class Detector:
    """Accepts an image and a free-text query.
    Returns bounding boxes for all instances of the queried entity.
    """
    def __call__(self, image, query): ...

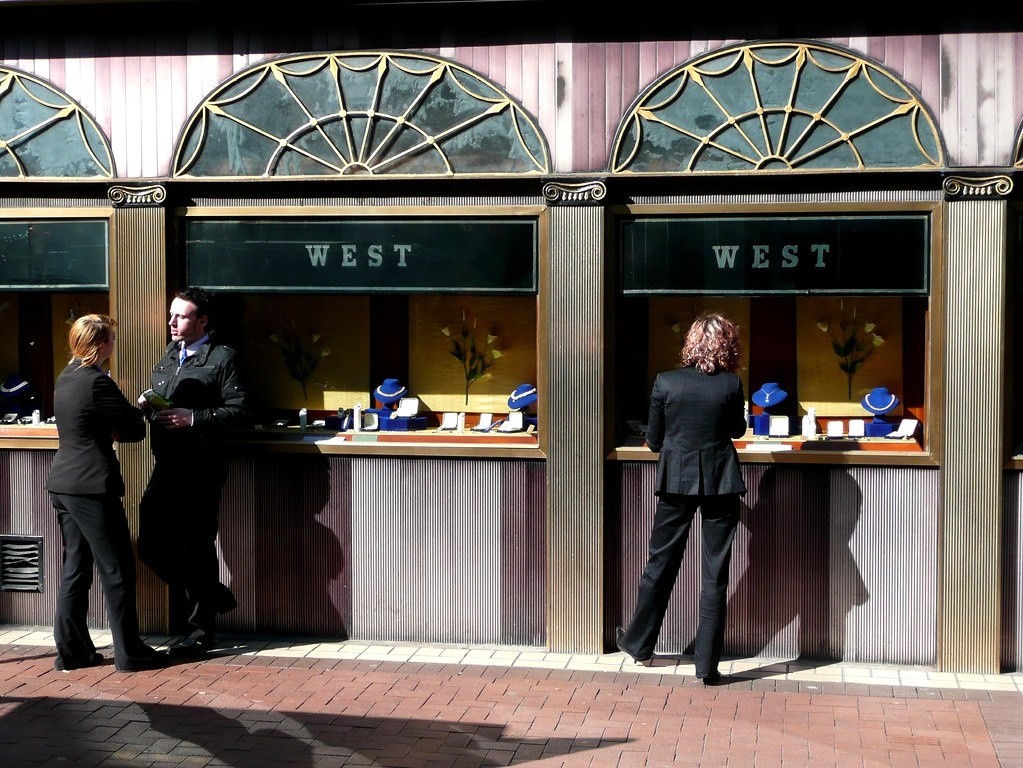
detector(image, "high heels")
[615,626,637,664]
[703,672,720,685]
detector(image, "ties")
[178,347,187,366]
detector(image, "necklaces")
[865,394,895,410]
[376,385,405,398]
[0,381,29,392]
[510,388,536,403]
[760,388,779,402]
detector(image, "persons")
[614,313,747,682]
[44,314,170,670]
[136,285,246,654]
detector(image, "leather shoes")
[214,584,237,616]
[168,632,214,657]
[201,635,203,637]
[86,653,103,665]
[144,651,167,670]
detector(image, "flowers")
[672,321,684,346]
[817,299,883,400]
[65,318,74,325]
[441,307,503,408]
[269,314,331,401]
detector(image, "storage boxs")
[497,411,523,433]
[396,397,419,417]
[768,414,790,438]
[440,413,458,430]
[884,418,920,439]
[361,413,380,431]
[849,418,865,439]
[825,419,845,440]
[0,413,18,424]
[470,413,493,432]
